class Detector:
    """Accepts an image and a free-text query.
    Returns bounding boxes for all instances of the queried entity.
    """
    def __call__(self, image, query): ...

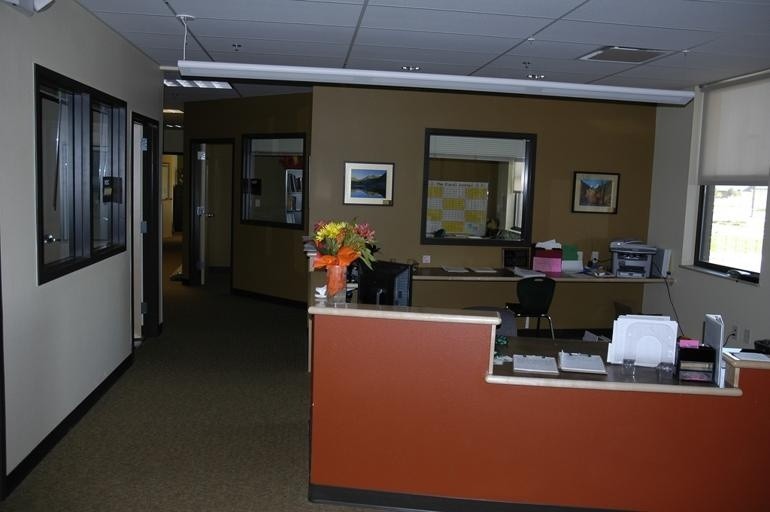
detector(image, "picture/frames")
[343,161,394,206]
[570,169,621,215]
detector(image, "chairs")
[505,276,558,338]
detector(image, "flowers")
[313,216,382,272]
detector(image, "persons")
[482,217,501,239]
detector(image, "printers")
[608,239,657,278]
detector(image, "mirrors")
[420,126,538,247]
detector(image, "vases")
[325,265,347,307]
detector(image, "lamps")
[176,11,697,108]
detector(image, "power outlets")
[731,326,738,341]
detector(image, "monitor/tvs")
[360,262,413,306]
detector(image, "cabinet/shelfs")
[285,169,304,223]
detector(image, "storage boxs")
[674,342,716,386]
[532,257,562,273]
[533,247,563,260]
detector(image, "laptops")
[503,247,530,270]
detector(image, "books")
[287,174,302,224]
[470,266,497,273]
[661,248,671,275]
[651,247,667,278]
[442,265,469,273]
[514,268,546,279]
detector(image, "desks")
[307,300,770,511]
